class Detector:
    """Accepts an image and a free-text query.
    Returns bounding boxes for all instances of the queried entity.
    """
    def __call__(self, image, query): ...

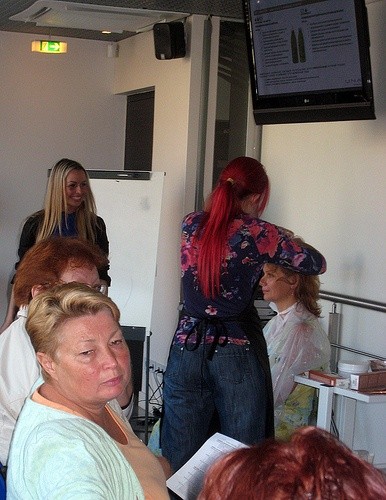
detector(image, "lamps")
[106,41,119,59]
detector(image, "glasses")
[83,282,107,295]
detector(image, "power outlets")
[148,359,165,379]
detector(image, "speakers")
[153,22,187,60]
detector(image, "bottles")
[337,359,369,379]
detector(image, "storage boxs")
[306,368,386,390]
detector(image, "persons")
[0,158,112,335]
[159,157,326,500]
[198,427,386,500]
[148,259,332,461]
[0,239,102,500]
[5,283,173,500]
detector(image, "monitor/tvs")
[242,0,377,125]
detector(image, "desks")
[293,373,386,434]
